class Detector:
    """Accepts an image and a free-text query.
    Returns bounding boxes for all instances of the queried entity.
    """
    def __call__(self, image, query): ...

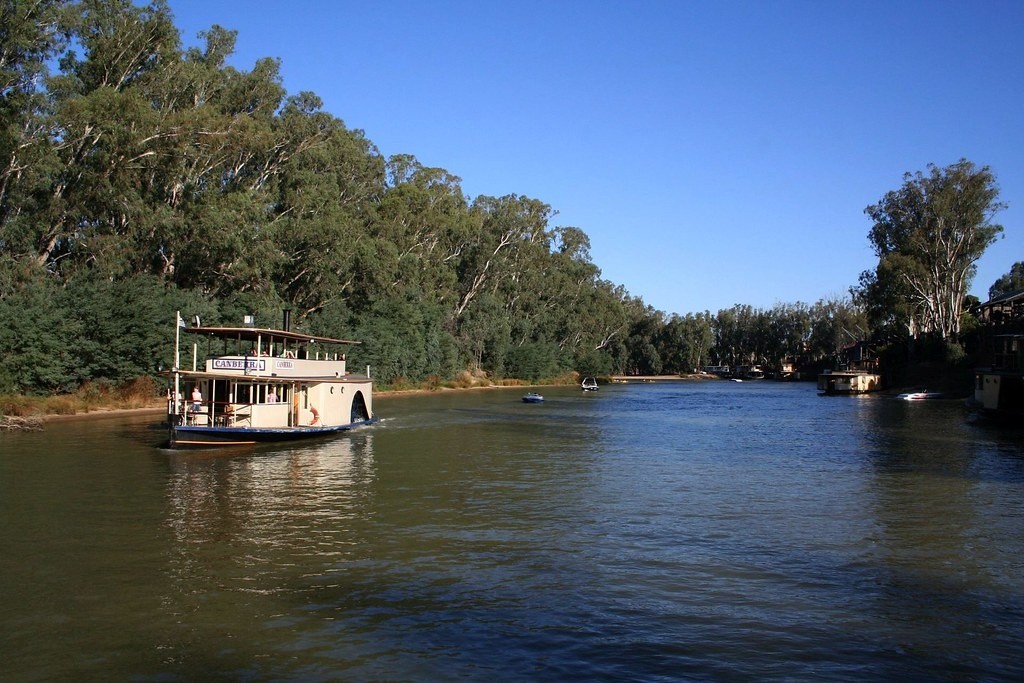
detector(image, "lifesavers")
[309,407,318,427]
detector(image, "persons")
[191,385,203,411]
[338,353,344,360]
[249,346,306,359]
[268,388,279,402]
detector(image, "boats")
[816,340,888,393]
[521,393,545,403]
[166,309,378,445]
[728,378,743,383]
[897,393,943,401]
[581,377,601,391]
